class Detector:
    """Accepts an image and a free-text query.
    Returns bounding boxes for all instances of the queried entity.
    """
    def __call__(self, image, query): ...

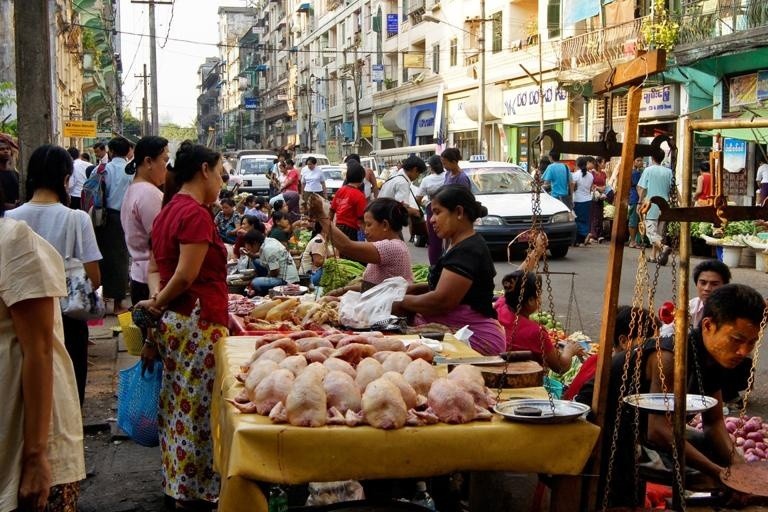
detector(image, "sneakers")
[578,233,605,248]
[630,239,672,265]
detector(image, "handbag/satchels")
[412,210,428,235]
[80,163,108,227]
[116,359,163,448]
[59,210,106,320]
[117,311,143,356]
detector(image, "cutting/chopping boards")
[450,360,545,388]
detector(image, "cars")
[408,154,577,259]
[293,144,437,199]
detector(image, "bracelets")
[144,339,159,348]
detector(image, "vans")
[223,150,278,194]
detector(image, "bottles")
[268,482,289,512]
[412,481,435,511]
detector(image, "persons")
[626,147,674,267]
[217,148,472,306]
[533,153,608,248]
[534,303,661,512]
[131,137,230,512]
[494,224,586,379]
[0,202,85,512]
[690,163,712,207]
[384,181,507,354]
[572,281,766,511]
[659,258,757,419]
[2,141,100,427]
[59,134,130,314]
[755,153,768,205]
[0,131,20,213]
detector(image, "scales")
[719,304,768,497]
[622,196,768,413]
[273,211,310,294]
[492,128,673,423]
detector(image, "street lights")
[422,14,484,148]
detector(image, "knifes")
[434,351,534,366]
